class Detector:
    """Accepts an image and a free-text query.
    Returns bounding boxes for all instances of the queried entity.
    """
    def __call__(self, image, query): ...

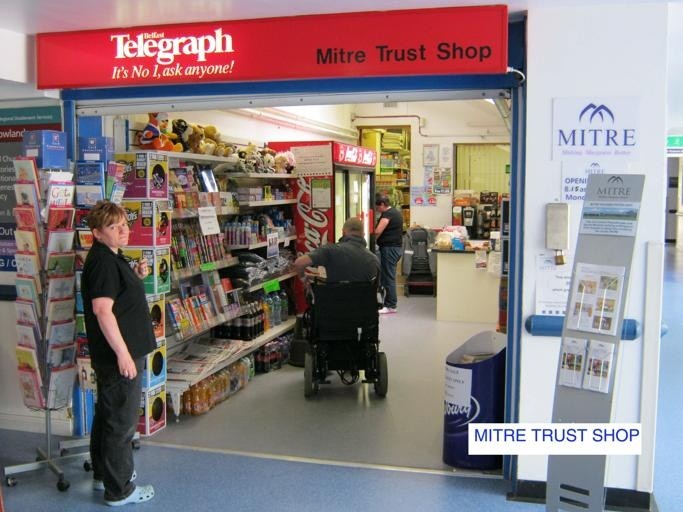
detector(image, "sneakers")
[378,307,397,313]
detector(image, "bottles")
[224,220,251,244]
[180,333,294,415]
[216,288,288,340]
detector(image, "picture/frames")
[423,144,439,166]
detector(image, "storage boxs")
[112,152,171,438]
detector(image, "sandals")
[104,484,154,506]
[93,469,137,490]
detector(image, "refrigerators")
[268,140,378,317]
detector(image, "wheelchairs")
[303,264,388,398]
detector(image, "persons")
[82,200,153,506]
[293,216,379,384]
[375,194,401,309]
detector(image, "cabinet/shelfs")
[114,119,309,424]
[359,125,411,235]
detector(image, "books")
[379,132,404,150]
[8,128,128,408]
[165,269,256,385]
